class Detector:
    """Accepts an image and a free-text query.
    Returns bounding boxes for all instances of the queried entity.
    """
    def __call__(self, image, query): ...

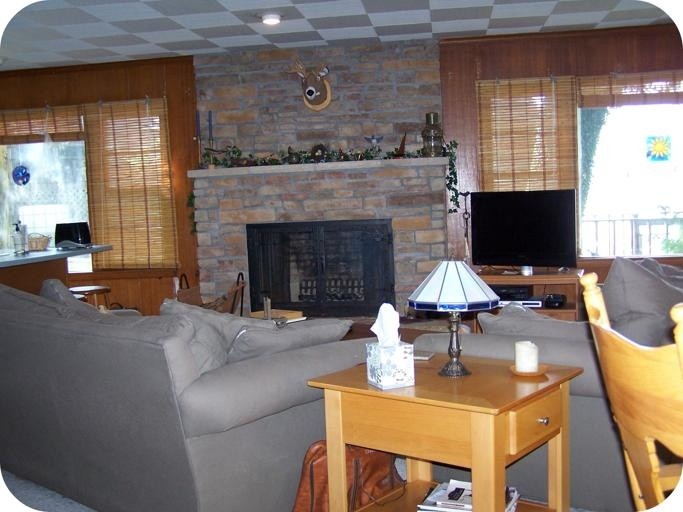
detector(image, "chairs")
[578,270,683,512]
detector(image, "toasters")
[54,222,91,249]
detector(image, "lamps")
[406,257,503,379]
[260,13,284,28]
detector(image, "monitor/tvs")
[471,190,576,276]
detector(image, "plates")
[509,364,546,376]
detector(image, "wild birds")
[364,134,383,148]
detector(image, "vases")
[421,112,445,159]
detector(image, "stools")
[69,285,111,310]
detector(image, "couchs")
[412,255,683,511]
[1,281,378,512]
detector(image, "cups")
[521,265,533,276]
[513,341,540,372]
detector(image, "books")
[416,479,520,512]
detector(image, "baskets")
[28,236,49,251]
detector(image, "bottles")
[422,111,443,158]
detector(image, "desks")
[1,243,114,296]
[306,349,585,512]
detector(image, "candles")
[513,340,542,376]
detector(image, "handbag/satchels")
[293,441,405,512]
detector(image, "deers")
[291,59,329,105]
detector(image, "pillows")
[158,294,277,354]
[476,254,683,340]
[226,317,356,364]
[39,274,99,320]
